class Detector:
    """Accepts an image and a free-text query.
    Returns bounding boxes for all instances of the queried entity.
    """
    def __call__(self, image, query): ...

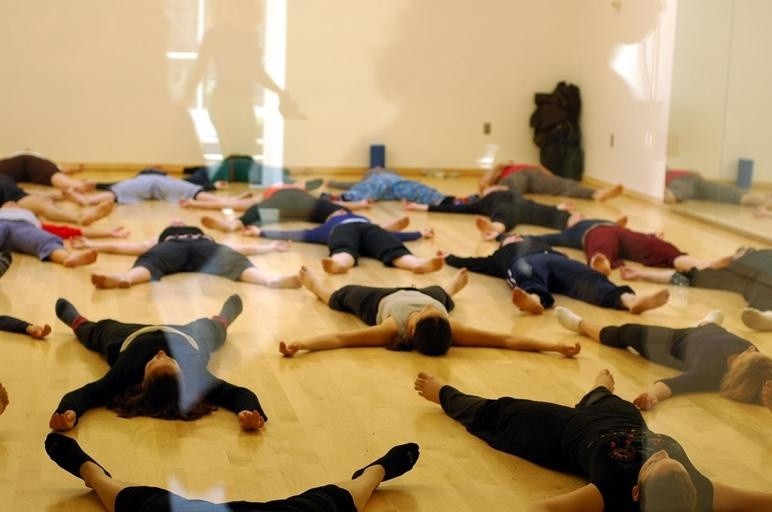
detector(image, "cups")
[370,144,385,169]
[736,159,753,187]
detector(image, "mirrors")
[662,0,771,240]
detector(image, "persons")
[0,200,130,267]
[0,383,9,416]
[751,205,772,218]
[479,213,733,277]
[414,369,772,512]
[243,208,446,275]
[181,156,323,192]
[0,175,113,226]
[179,184,374,233]
[402,186,584,235]
[551,307,772,412]
[63,168,253,206]
[50,294,268,430]
[663,167,765,204]
[435,234,670,315]
[479,160,623,202]
[45,433,419,512]
[279,266,581,358]
[0,155,96,193]
[619,248,772,333]
[69,220,302,288]
[321,165,480,205]
[0,250,51,339]
